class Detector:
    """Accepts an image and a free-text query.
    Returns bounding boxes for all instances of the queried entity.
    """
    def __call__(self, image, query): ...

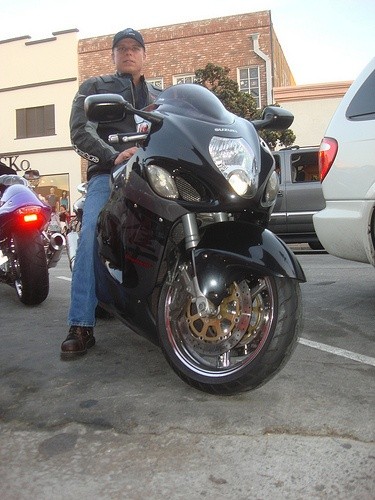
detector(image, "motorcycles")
[0,169,66,306]
[66,83,308,396]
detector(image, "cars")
[267,56,374,266]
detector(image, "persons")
[60,190,70,224]
[59,28,164,359]
[59,205,68,234]
[45,186,59,213]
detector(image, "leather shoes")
[59,327,95,359]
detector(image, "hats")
[112,29,144,48]
